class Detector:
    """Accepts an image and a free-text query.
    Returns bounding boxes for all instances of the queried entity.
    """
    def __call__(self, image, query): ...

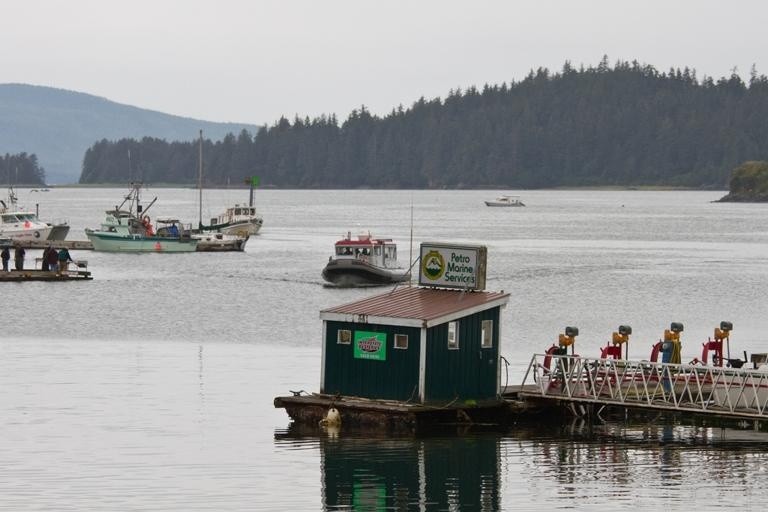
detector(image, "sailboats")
[182,129,264,251]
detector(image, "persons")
[1,244,10,271]
[15,243,25,269]
[40,246,72,272]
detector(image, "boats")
[321,228,412,287]
[484,193,527,207]
[533,321,768,411]
[206,203,263,234]
[86,177,195,251]
[0,181,71,239]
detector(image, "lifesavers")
[651,342,663,382]
[544,346,566,389]
[600,347,620,386]
[702,342,722,365]
[144,215,150,223]
